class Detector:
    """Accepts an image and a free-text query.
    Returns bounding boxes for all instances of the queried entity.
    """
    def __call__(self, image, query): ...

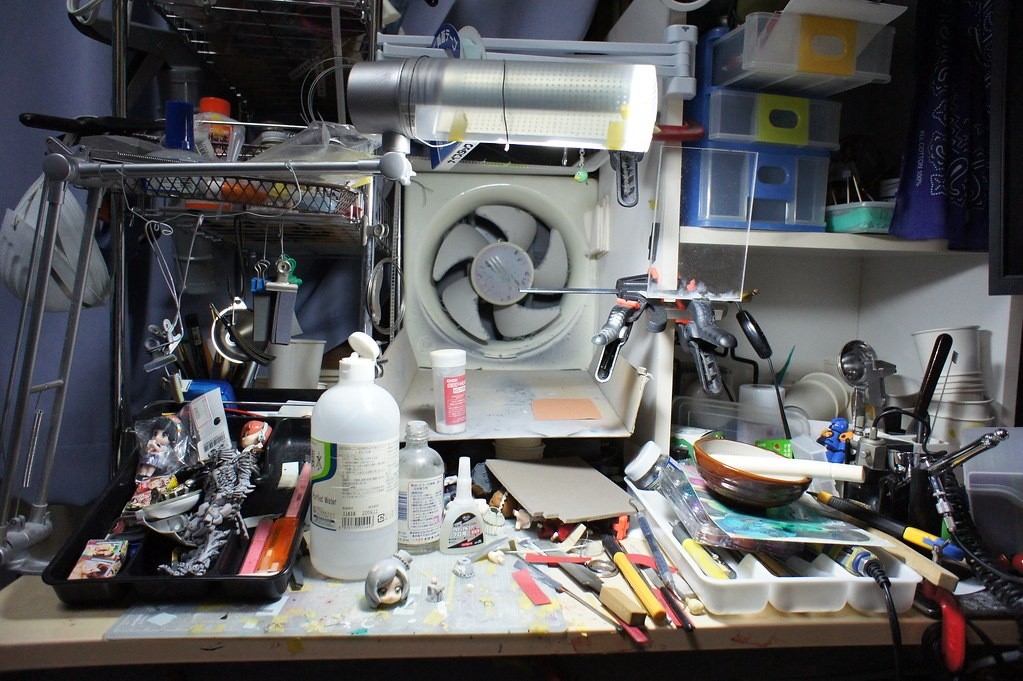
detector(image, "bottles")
[625,440,682,490]
[399,422,446,555]
[309,331,400,581]
[193,98,245,163]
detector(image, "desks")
[0,427,1023,681]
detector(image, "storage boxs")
[683,11,895,233]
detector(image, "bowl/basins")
[694,437,812,505]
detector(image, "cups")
[884,325,997,446]
[493,438,545,460]
[431,349,467,433]
[784,371,849,422]
[268,338,328,390]
